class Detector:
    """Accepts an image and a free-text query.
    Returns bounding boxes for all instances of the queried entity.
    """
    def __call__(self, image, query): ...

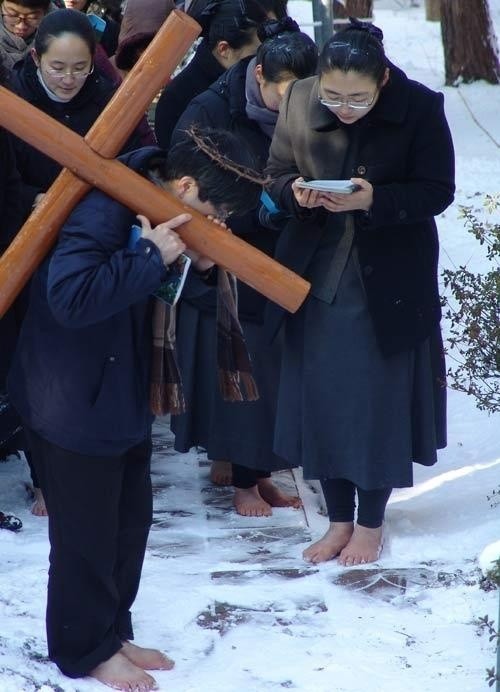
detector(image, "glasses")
[317,78,379,109]
[38,55,95,79]
[0,4,41,28]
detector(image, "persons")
[0,0,322,525]
[262,22,456,568]
[11,127,264,691]
